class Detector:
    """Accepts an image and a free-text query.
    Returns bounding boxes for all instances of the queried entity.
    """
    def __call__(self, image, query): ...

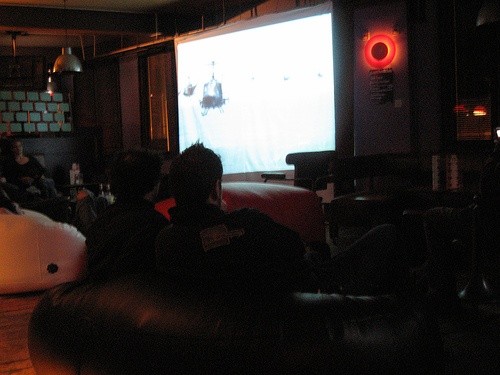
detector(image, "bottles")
[98,183,113,204]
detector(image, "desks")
[63,183,97,194]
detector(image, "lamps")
[51,0,84,75]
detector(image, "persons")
[83,161,169,287]
[0,137,56,214]
[151,143,313,312]
[324,187,436,325]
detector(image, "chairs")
[321,152,416,247]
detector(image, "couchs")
[28,273,447,375]
